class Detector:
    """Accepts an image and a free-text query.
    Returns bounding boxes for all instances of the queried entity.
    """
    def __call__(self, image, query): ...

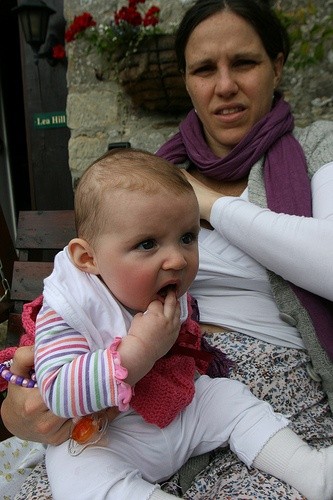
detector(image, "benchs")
[6,211,79,347]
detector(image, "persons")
[0,0,333,500]
[34,148,333,500]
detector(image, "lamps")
[12,0,59,68]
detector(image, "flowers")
[53,0,176,111]
